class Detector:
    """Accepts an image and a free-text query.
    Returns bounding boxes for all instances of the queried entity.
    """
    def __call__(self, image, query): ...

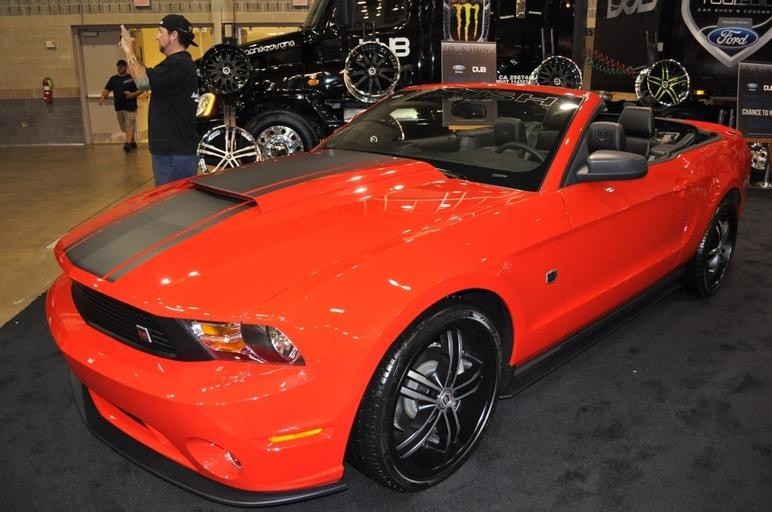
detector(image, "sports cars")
[37,80,758,510]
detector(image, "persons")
[118,14,201,188]
[99,60,146,152]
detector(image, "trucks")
[186,1,771,191]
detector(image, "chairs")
[572,122,626,171]
[481,117,529,159]
[534,105,578,150]
[617,107,658,157]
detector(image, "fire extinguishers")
[43,77,53,103]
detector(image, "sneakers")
[130,142,137,149]
[123,141,130,152]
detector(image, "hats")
[158,14,199,48]
[116,59,127,65]
